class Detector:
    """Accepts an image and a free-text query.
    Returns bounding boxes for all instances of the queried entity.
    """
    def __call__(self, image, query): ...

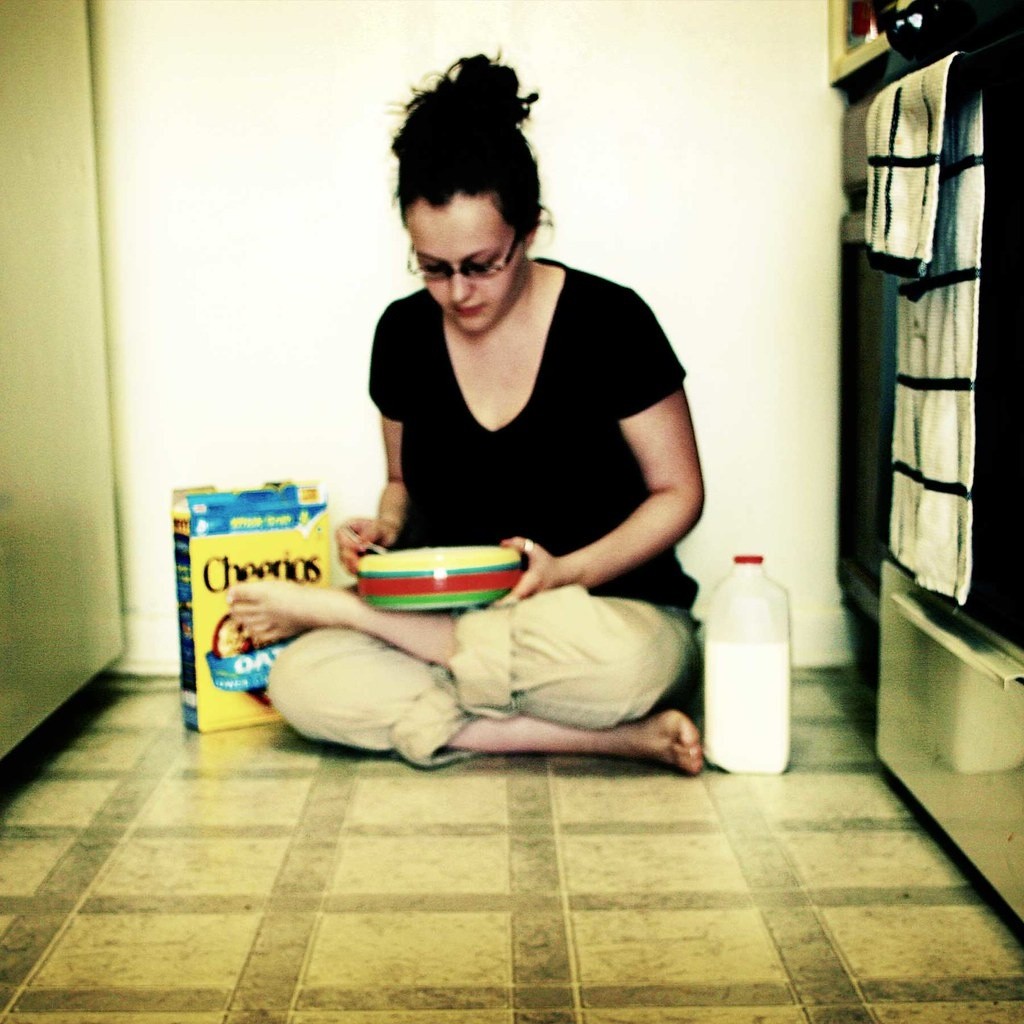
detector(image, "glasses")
[407,233,522,279]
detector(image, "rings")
[520,538,533,556]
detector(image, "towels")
[864,53,985,603]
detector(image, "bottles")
[705,555,790,775]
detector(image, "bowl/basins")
[356,546,522,612]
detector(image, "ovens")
[872,34,1024,946]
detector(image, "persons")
[228,53,706,776]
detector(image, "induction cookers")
[884,0,1024,59]
